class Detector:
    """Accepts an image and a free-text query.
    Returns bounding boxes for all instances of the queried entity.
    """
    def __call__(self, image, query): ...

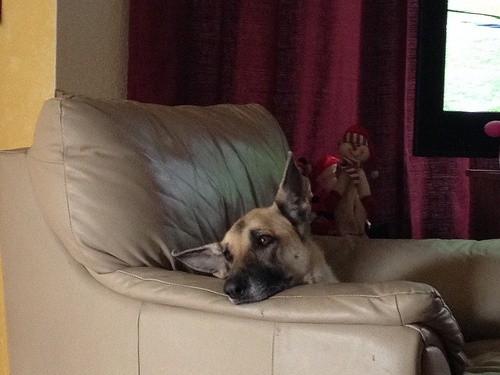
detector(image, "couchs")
[0,94,500,375]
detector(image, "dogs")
[172,151,341,305]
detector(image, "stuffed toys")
[314,127,379,236]
